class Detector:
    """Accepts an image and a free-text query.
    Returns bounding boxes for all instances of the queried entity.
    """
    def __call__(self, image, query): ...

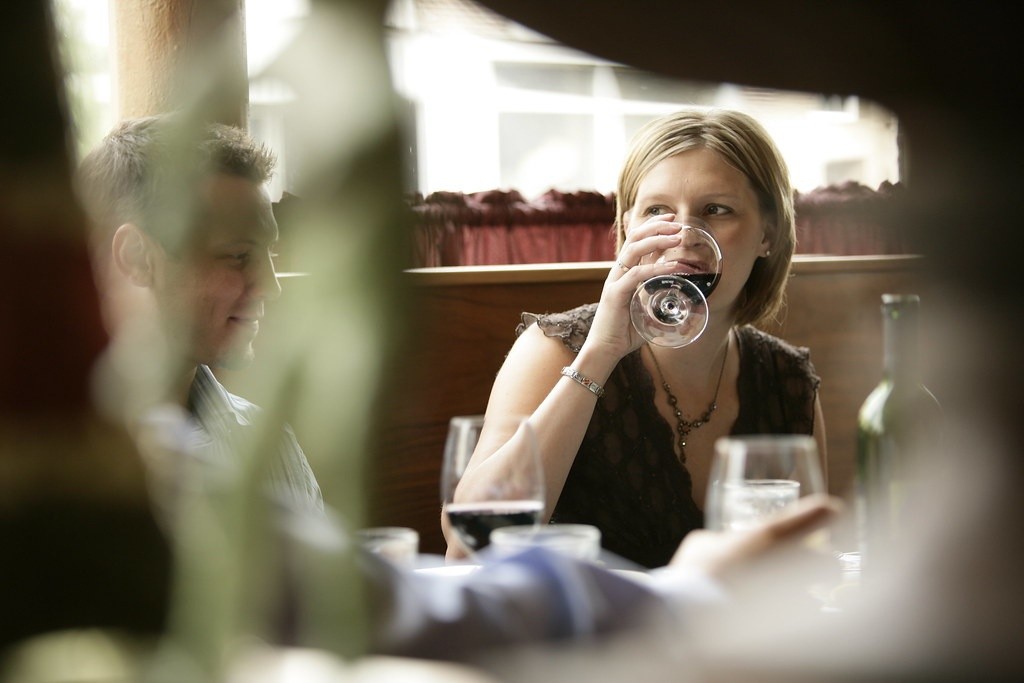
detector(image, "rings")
[617,260,629,273]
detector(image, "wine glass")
[630,226,724,349]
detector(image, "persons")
[441,108,832,565]
[80,117,845,660]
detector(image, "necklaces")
[646,336,731,465]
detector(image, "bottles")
[857,294,943,576]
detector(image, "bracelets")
[561,366,605,397]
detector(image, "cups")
[490,523,602,565]
[438,416,546,570]
[357,526,419,574]
[703,434,826,534]
